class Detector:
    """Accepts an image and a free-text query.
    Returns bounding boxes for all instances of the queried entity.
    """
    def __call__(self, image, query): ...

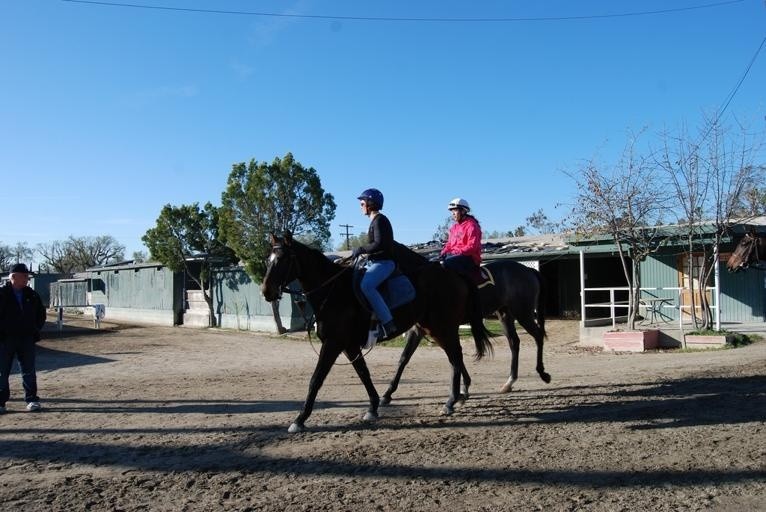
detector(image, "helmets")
[448,198,470,212]
[357,188,384,206]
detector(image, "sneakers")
[374,320,398,337]
[26,401,40,411]
[0,405,7,414]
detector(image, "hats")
[9,263,34,275]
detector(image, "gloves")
[352,246,365,258]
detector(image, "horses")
[378,240,551,406]
[726,231,766,275]
[259,227,504,433]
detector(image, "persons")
[353,188,400,337]
[0,262,46,414]
[440,198,481,284]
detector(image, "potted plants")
[684,330,742,351]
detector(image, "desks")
[639,298,675,326]
